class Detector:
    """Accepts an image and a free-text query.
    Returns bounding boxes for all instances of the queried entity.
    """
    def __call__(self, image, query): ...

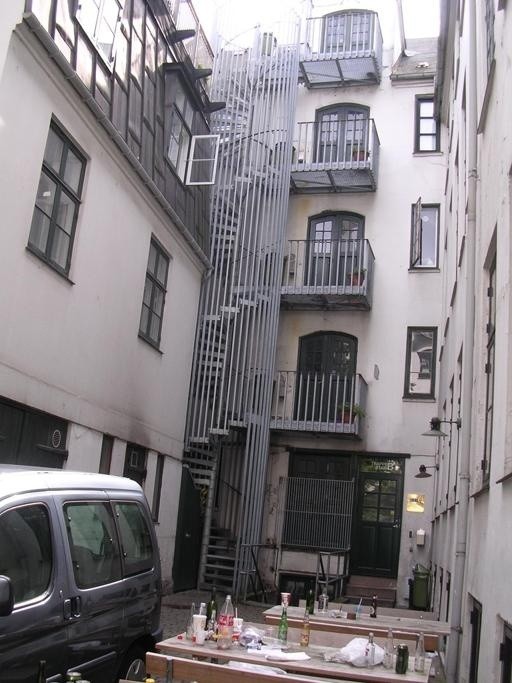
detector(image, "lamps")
[420,414,462,437]
[414,463,440,478]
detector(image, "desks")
[263,604,451,652]
[156,630,432,682]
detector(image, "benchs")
[298,600,440,622]
[266,616,439,654]
[144,652,310,683]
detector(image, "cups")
[232,616,243,632]
[353,604,364,619]
[194,625,209,645]
[280,592,292,608]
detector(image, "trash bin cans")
[412,563,429,609]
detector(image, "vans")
[1,460,165,683]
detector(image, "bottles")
[304,579,315,614]
[414,631,425,673]
[299,610,311,645]
[369,594,377,618]
[395,643,409,674]
[216,592,235,648]
[318,586,329,613]
[277,605,289,644]
[382,624,394,668]
[185,600,216,637]
[363,631,375,670]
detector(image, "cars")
[313,474,397,523]
